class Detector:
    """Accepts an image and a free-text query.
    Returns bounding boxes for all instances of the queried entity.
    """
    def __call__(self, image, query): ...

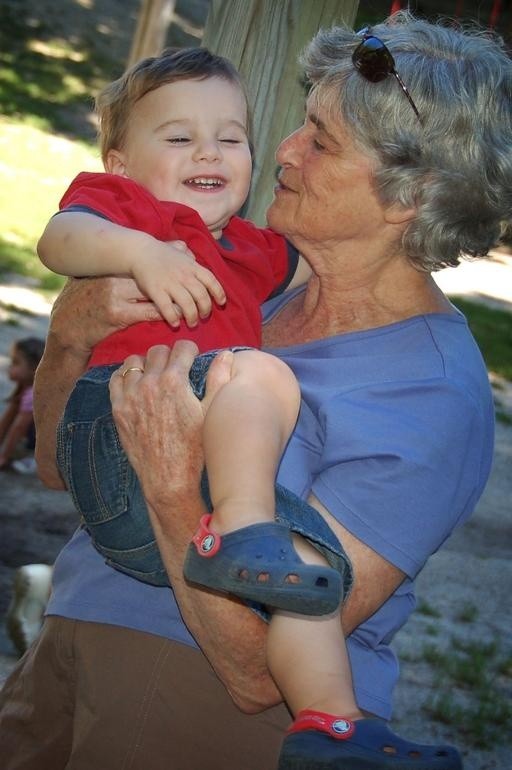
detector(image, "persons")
[3,11,510,768]
[0,336,49,476]
[35,48,467,768]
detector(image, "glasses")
[352,26,424,126]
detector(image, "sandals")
[280,710,463,770]
[182,513,344,616]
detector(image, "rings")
[122,366,144,378]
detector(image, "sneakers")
[12,458,37,474]
[6,563,50,652]
